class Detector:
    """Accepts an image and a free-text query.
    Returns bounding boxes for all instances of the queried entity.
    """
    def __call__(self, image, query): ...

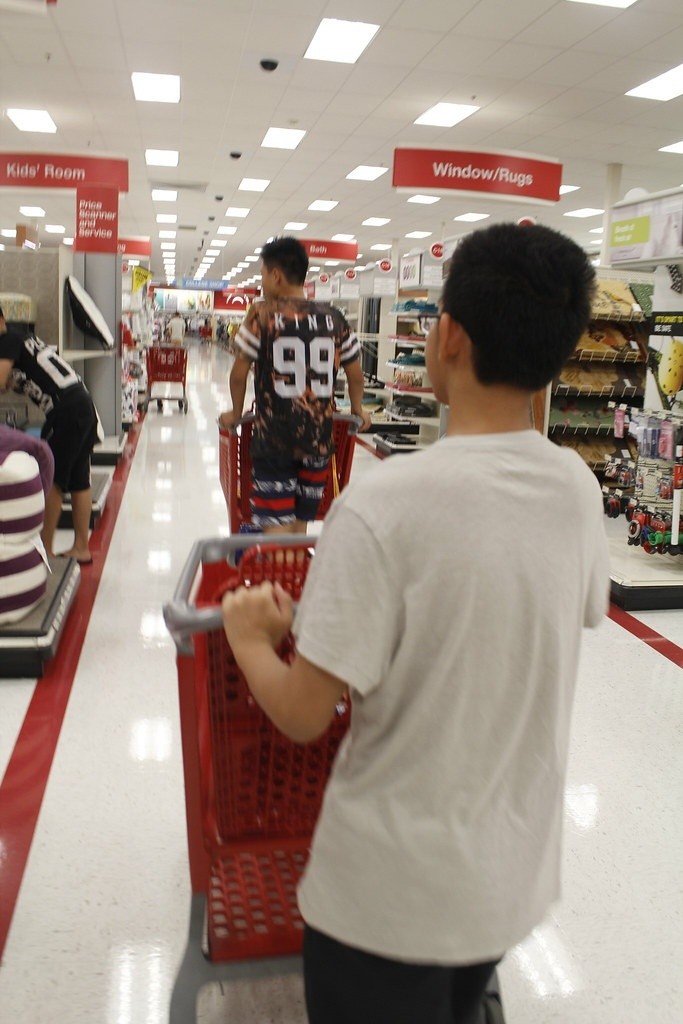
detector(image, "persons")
[0,308,107,567]
[168,312,187,347]
[221,221,612,1024]
[218,235,372,562]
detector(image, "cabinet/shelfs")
[57,246,168,466]
[531,268,652,499]
[319,284,447,461]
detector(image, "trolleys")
[167,537,359,963]
[144,340,190,414]
[219,406,363,539]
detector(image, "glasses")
[417,311,442,332]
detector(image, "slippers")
[56,551,94,564]
